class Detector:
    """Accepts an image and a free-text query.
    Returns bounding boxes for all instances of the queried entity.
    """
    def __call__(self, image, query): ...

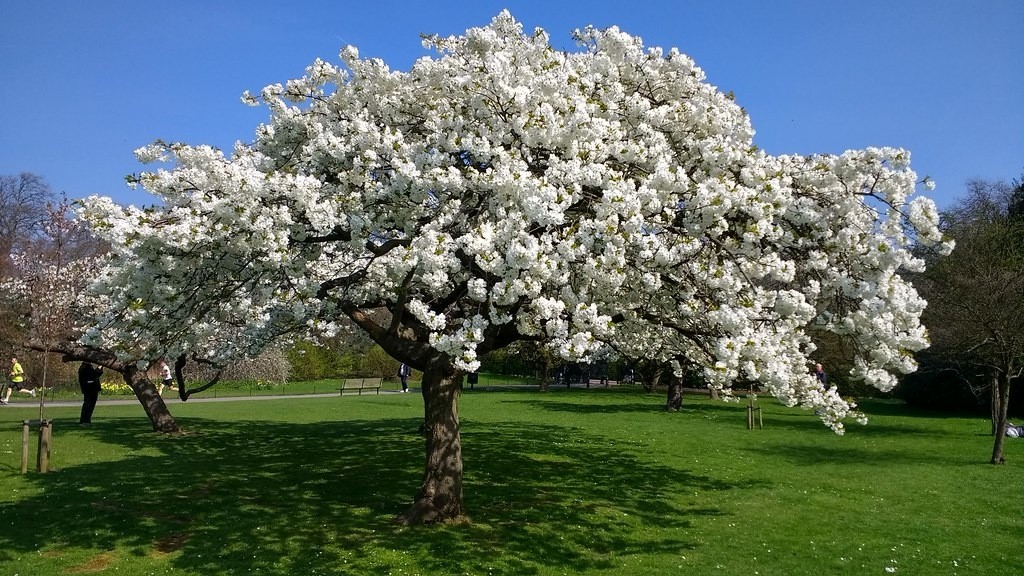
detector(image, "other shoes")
[0,399,8,404]
[406,388,408,392]
[400,390,404,393]
[32,389,36,398]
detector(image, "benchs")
[360,378,383,394]
[335,379,363,396]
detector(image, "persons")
[811,363,826,415]
[78,361,103,424]
[555,372,589,386]
[0,357,37,405]
[158,361,179,396]
[396,363,409,393]
